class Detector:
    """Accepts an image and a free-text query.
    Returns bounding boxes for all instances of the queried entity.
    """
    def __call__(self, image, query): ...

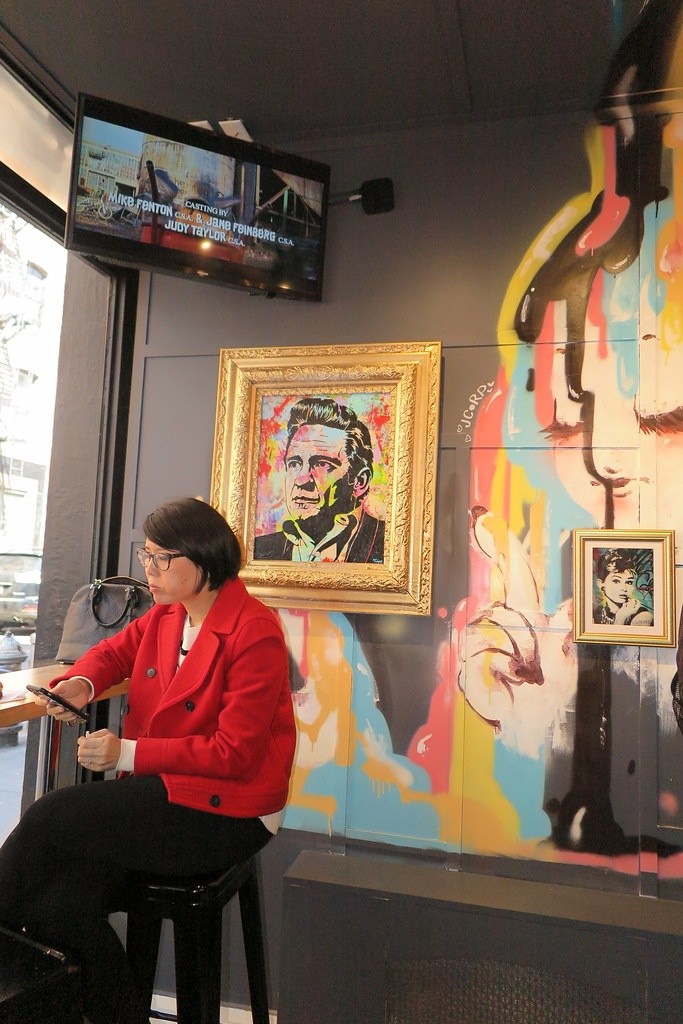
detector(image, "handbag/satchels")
[54,576,156,665]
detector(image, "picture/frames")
[572,529,677,648]
[209,341,442,616]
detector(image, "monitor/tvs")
[64,91,330,305]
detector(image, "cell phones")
[26,685,88,722]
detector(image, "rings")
[87,757,91,764]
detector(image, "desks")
[0,663,130,794]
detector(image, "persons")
[0,497,297,1024]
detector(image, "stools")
[127,853,268,1024]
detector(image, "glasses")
[137,547,187,571]
